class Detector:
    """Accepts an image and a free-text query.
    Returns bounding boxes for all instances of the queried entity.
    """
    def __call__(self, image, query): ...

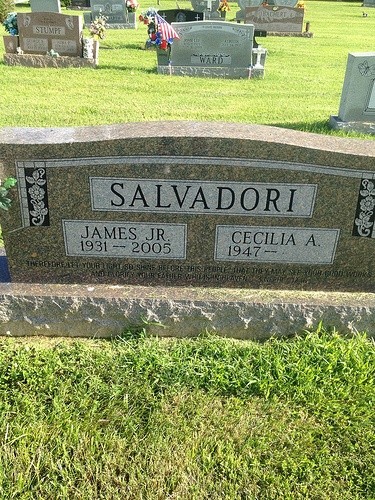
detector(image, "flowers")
[88,8,113,39]
[217,0,230,15]
[126,0,138,13]
[2,12,18,37]
[139,7,171,52]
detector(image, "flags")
[154,13,180,45]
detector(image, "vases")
[3,35,19,54]
[82,39,94,59]
[128,13,135,23]
[157,50,170,66]
[221,11,226,18]
